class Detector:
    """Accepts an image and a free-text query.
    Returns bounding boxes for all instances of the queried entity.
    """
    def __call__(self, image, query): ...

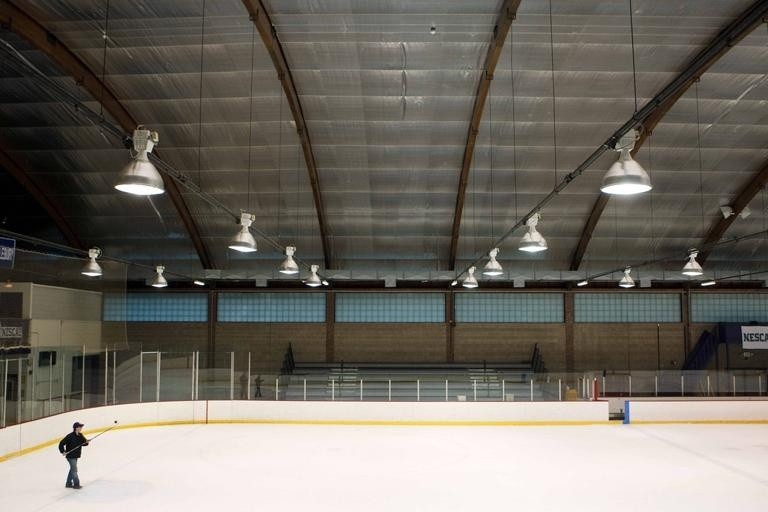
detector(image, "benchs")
[286,360,546,403]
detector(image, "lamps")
[113,125,322,290]
[79,247,171,290]
[739,207,752,220]
[460,119,655,292]
[720,206,735,220]
[617,248,705,289]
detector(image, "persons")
[254,373,264,398]
[58,422,91,489]
[240,370,248,399]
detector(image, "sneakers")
[73,486,83,489]
[66,485,74,488]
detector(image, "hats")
[73,422,84,428]
